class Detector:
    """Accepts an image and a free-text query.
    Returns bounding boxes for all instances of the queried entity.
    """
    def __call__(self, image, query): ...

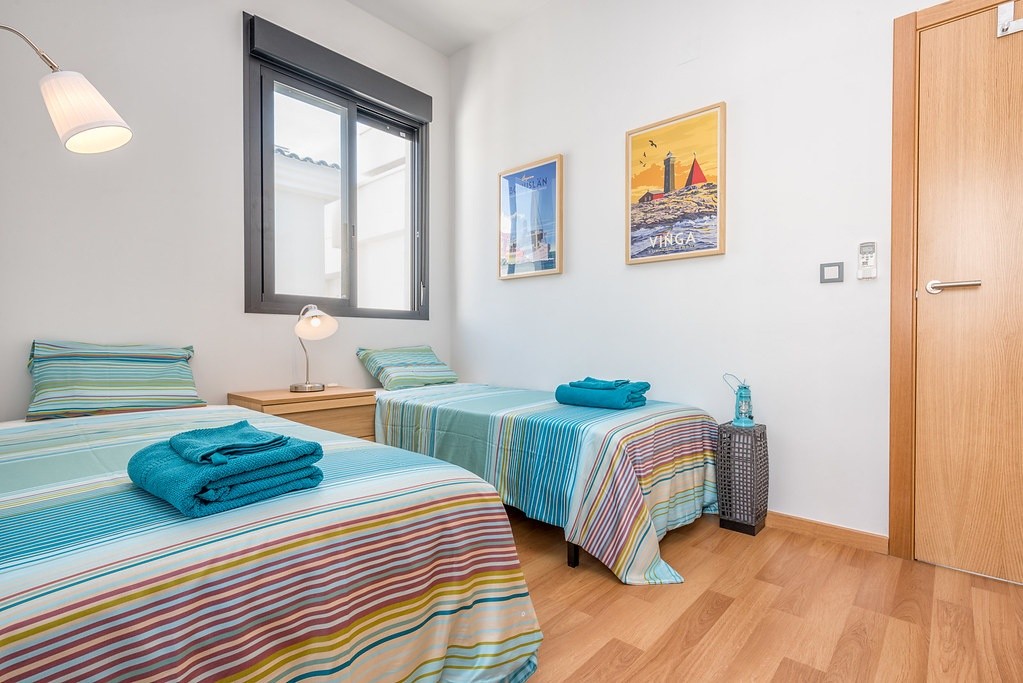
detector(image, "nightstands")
[227,387,378,444]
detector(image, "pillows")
[25,338,209,421]
[354,345,459,389]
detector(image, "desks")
[715,420,769,535]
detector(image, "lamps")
[289,304,339,393]
[722,373,756,430]
[0,26,133,153]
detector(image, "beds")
[1,410,544,683]
[368,384,721,584]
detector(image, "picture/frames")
[497,153,564,279]
[624,102,726,265]
